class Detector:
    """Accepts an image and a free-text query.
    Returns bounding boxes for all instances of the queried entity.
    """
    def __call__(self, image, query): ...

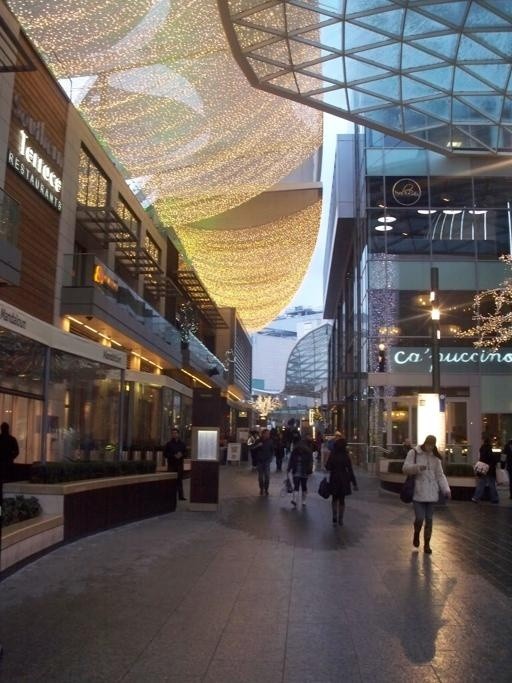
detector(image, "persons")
[325,430,359,524]
[400,435,451,553]
[162,427,186,501]
[0,421,20,465]
[247,418,324,507]
[470,431,512,506]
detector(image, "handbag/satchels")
[284,479,293,493]
[318,476,331,499]
[473,460,490,476]
[400,479,416,503]
[496,463,511,486]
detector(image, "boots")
[291,490,308,506]
[413,522,432,553]
[333,510,344,526]
